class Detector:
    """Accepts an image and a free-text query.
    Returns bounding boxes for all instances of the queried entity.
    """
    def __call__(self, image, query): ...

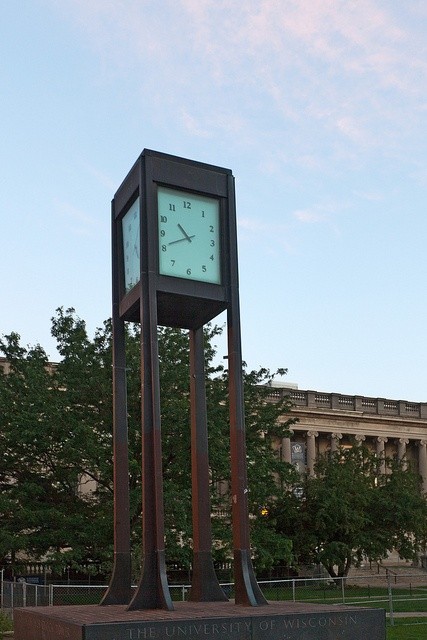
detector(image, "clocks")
[114,187,143,292]
[159,183,225,285]
